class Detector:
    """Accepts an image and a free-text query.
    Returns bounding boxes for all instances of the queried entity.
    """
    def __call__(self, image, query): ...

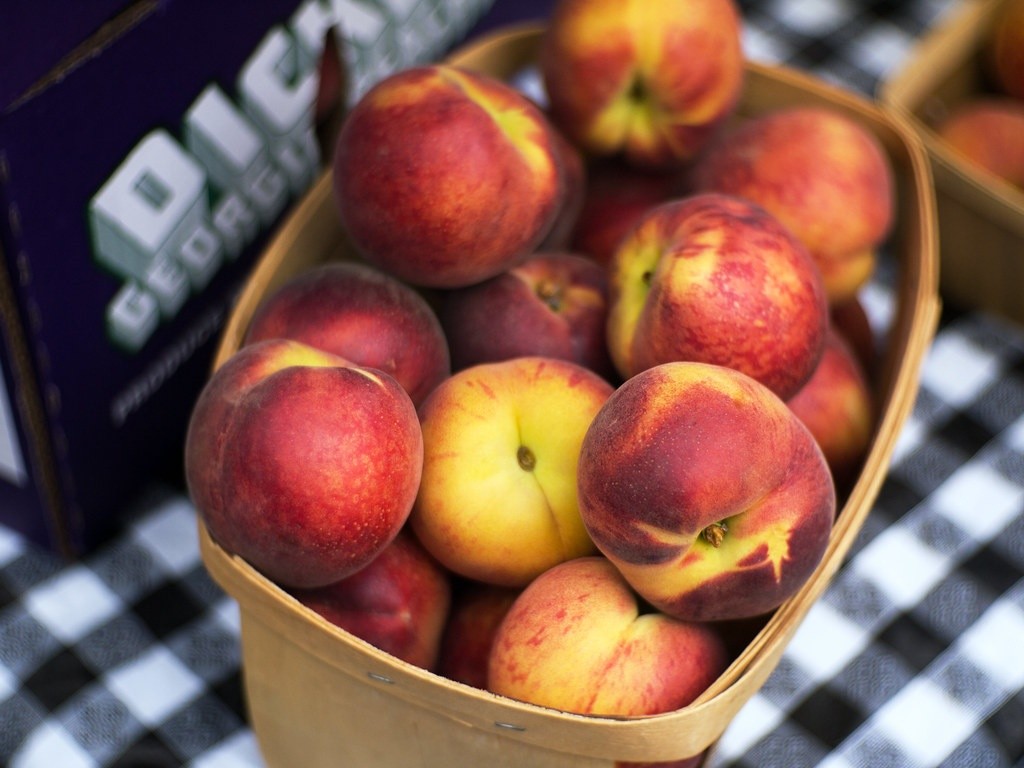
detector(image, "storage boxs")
[0,0,508,563]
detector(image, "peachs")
[929,1,1024,198]
[183,0,894,722]
